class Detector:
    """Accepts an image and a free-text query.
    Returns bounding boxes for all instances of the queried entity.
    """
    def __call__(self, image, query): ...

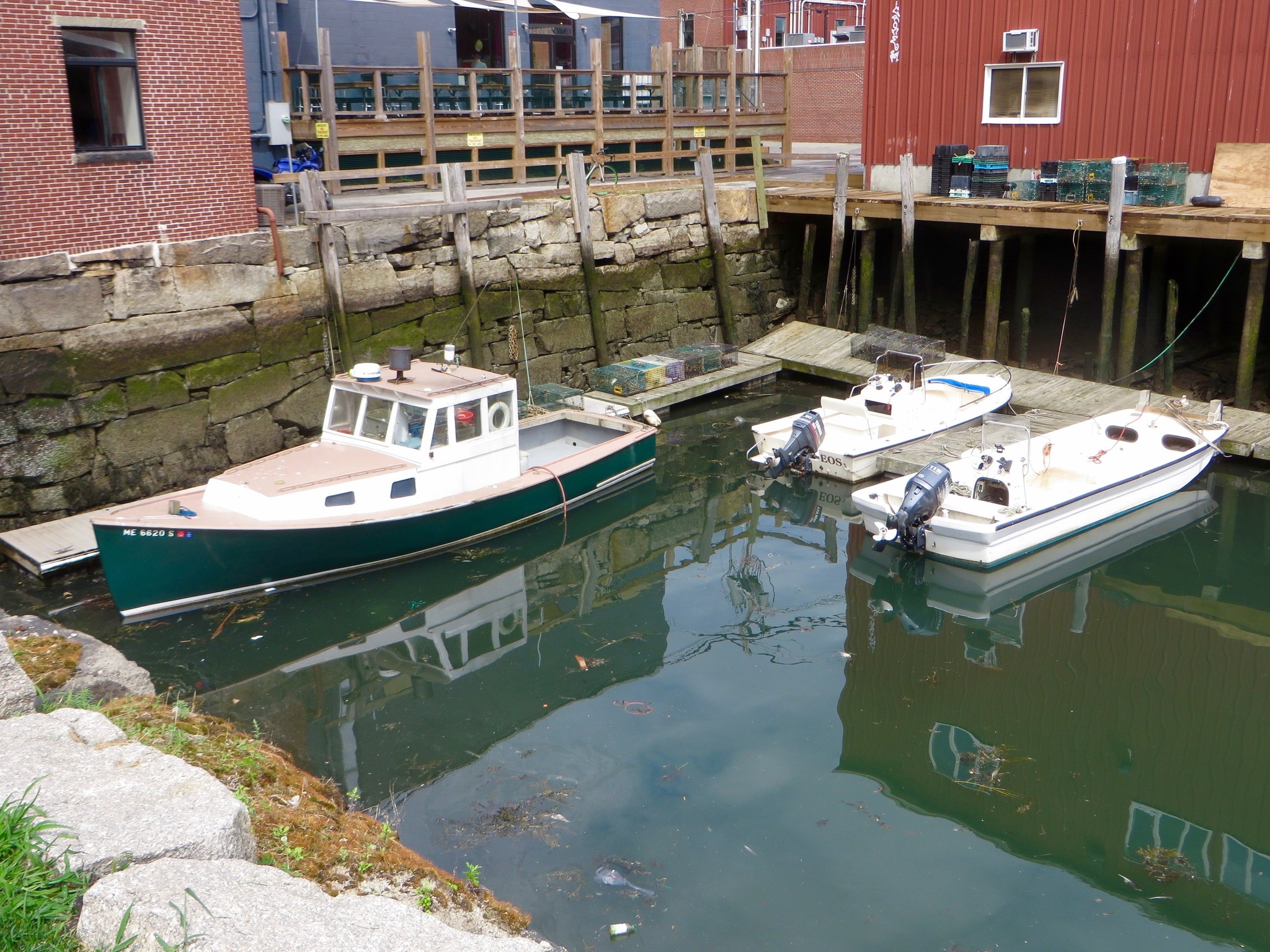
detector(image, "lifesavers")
[488,401,511,431]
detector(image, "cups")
[458,76,464,85]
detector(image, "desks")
[294,81,662,120]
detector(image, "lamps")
[581,26,588,35]
[522,22,528,33]
[448,27,456,34]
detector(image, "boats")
[746,350,1013,486]
[746,472,891,532]
[851,389,1230,570]
[847,489,1220,671]
[88,344,662,619]
[103,468,663,706]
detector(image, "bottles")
[1030,167,1035,180]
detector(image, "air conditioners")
[255,180,286,226]
[1003,30,1037,51]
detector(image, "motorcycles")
[252,143,333,211]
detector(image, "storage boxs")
[931,144,1160,205]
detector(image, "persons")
[462,49,487,86]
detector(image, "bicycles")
[557,147,618,200]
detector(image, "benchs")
[821,396,883,442]
